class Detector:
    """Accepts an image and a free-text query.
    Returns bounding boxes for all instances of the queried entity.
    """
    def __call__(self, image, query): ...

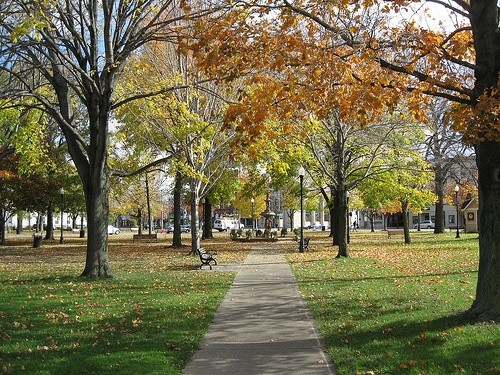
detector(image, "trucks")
[214,219,245,232]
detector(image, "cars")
[413,220,435,229]
[167,224,191,233]
[107,224,122,235]
[33,224,48,230]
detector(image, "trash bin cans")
[80,230,85,238]
[33,235,42,248]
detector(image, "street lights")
[59,187,65,244]
[250,198,255,232]
[346,191,352,243]
[298,165,309,251]
[453,186,461,238]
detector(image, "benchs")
[196,247,217,270]
[387,229,406,238]
[132,234,157,244]
[299,238,312,251]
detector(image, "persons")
[352,221,358,232]
